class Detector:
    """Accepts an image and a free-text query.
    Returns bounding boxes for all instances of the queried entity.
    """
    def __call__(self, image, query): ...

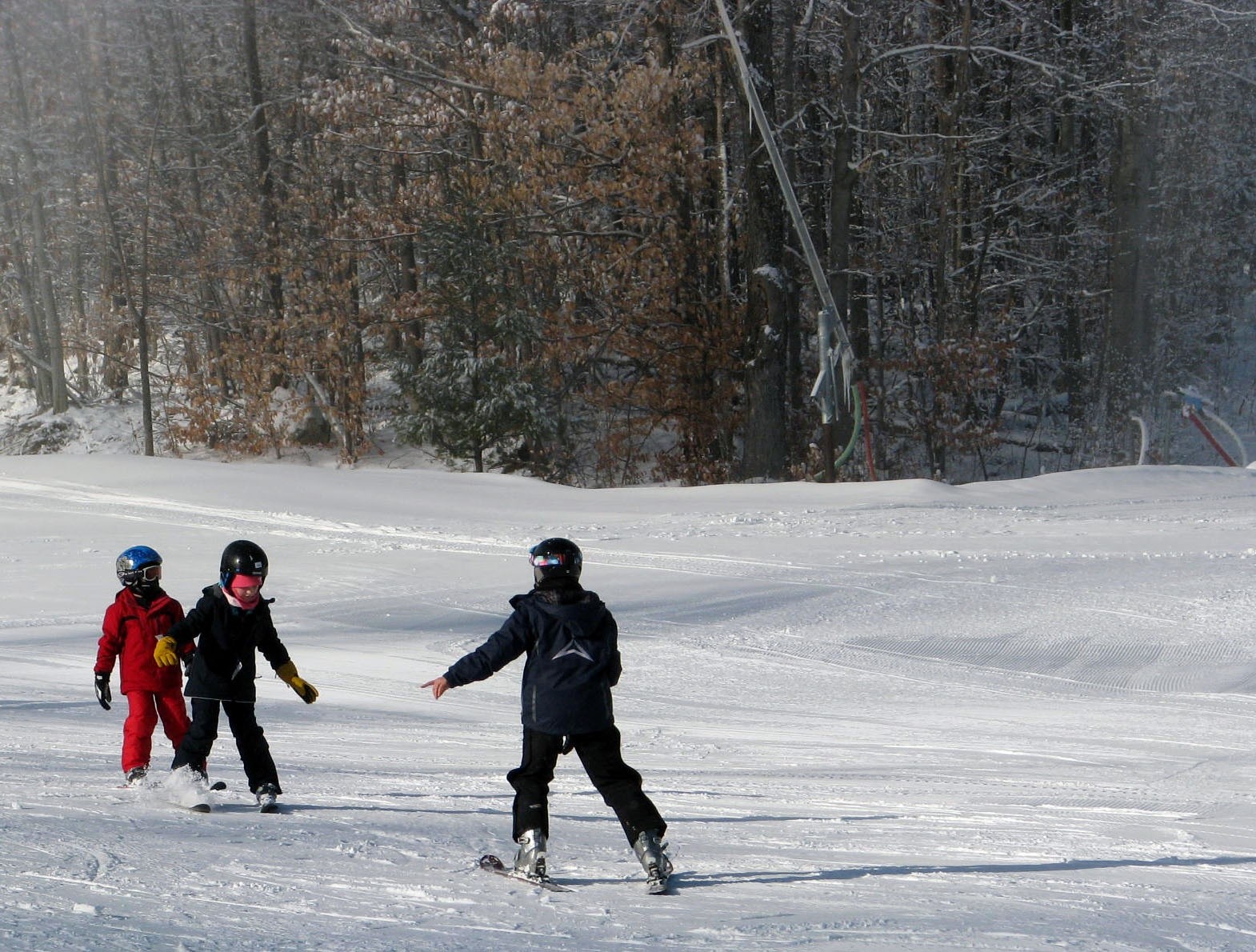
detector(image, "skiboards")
[477,851,676,896]
[119,774,229,788]
[169,790,282,814]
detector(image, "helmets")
[219,539,268,598]
[115,545,163,585]
[528,537,582,587]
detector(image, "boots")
[125,768,150,787]
[513,827,547,883]
[256,782,276,806]
[636,831,671,892]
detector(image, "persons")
[419,535,672,882]
[150,540,319,816]
[90,547,211,791]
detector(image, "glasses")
[142,565,162,580]
